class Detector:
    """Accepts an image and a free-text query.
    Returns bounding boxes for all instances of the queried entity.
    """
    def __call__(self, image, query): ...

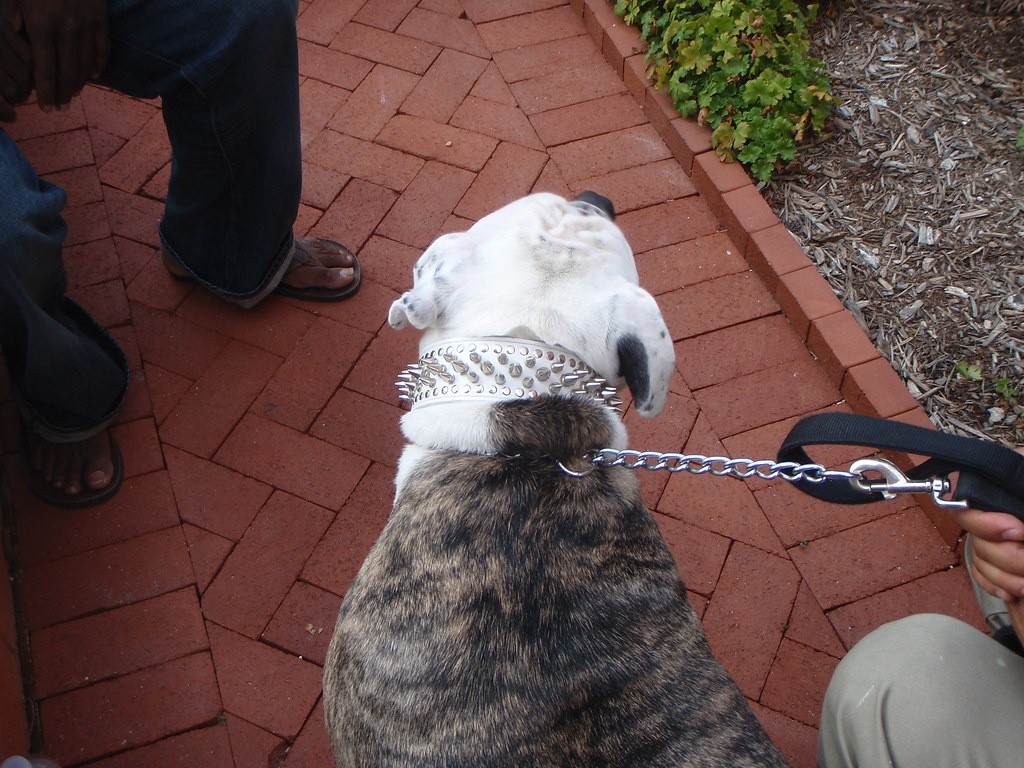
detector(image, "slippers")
[168,237,362,303]
[20,416,124,509]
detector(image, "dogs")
[318,189,797,768]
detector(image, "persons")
[0,0,360,511]
[817,446,1024,768]
